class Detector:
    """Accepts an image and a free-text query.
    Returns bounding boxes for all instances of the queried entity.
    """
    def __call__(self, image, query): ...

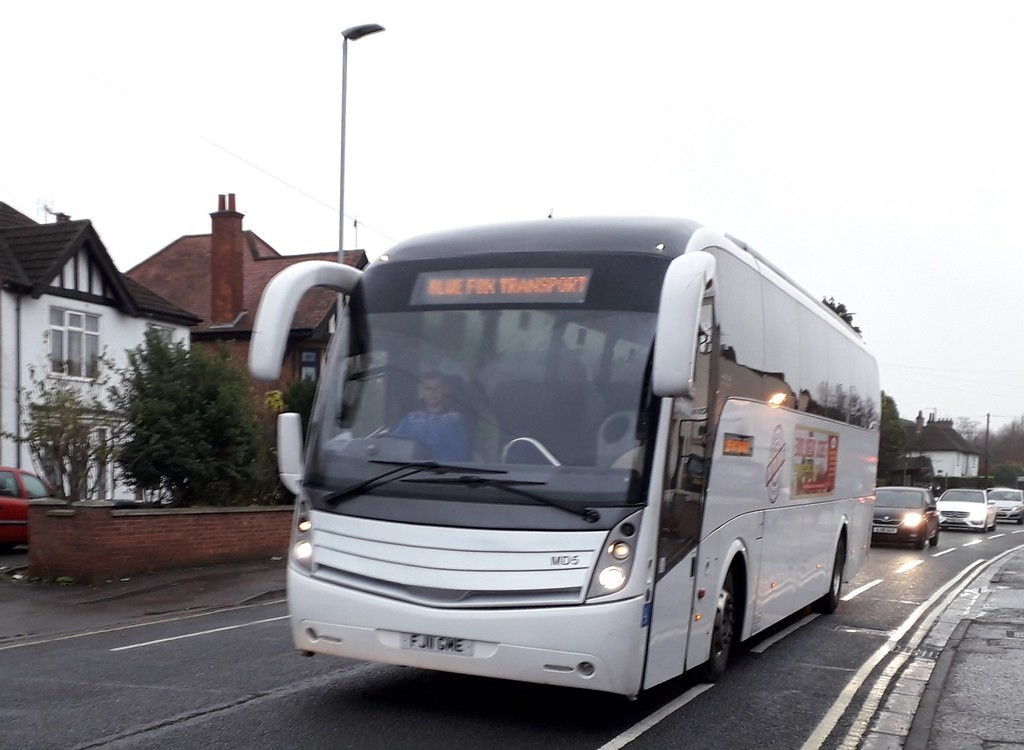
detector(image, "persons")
[393,376,472,460]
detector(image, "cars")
[868,485,942,550]
[986,488,1024,523]
[935,487,1001,534]
[1,464,56,556]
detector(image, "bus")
[246,214,883,707]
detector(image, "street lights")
[331,22,388,323]
